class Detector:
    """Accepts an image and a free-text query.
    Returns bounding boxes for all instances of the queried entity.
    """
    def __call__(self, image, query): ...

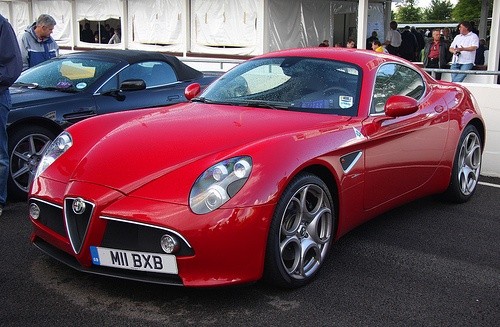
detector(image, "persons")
[0,14,24,220]
[79,23,121,44]
[319,21,491,70]
[17,15,60,70]
[449,21,478,82]
[423,28,448,81]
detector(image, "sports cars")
[8,49,249,200]
[25,44,489,294]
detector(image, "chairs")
[281,72,325,104]
